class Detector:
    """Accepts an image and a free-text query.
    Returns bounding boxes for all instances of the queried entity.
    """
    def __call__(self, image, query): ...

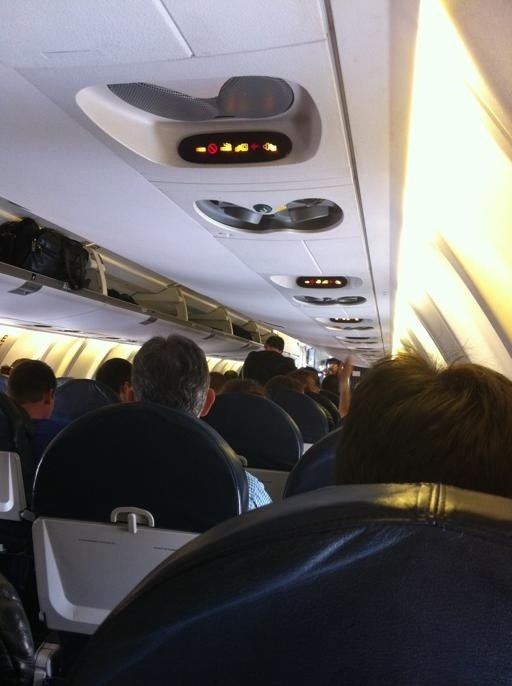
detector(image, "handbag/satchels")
[0,216,89,290]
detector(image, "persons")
[6,360,63,436]
[240,335,297,383]
[133,336,272,513]
[332,345,512,500]
[94,358,133,401]
[204,357,342,401]
